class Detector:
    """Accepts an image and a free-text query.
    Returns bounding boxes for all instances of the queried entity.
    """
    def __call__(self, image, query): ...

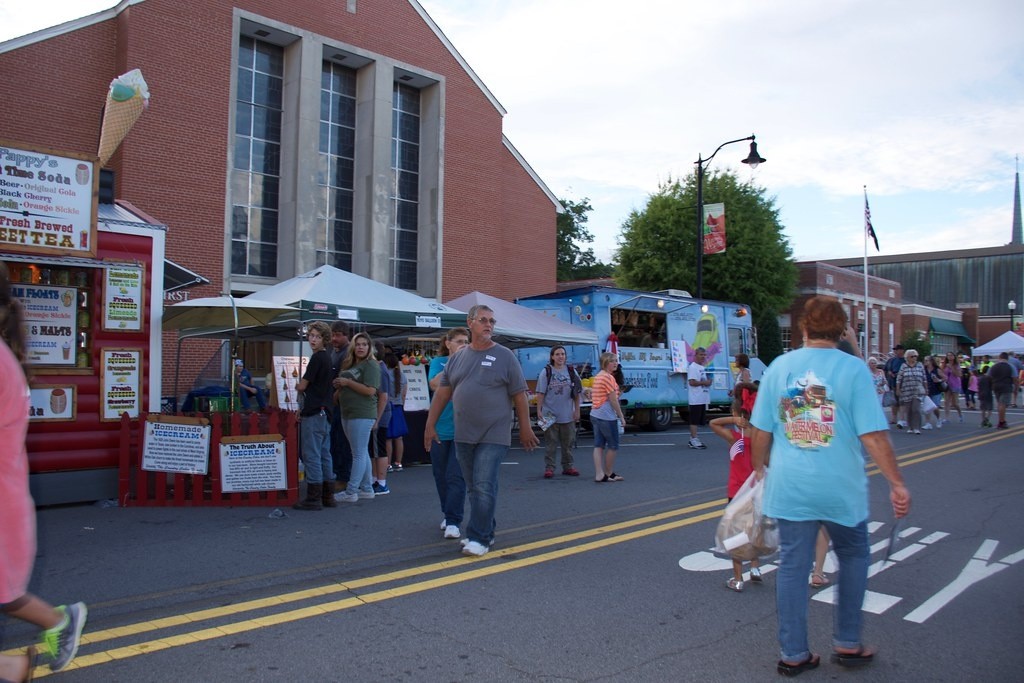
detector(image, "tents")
[439,288,601,351]
[971,330,1024,367]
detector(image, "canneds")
[20,267,88,287]
[77,290,89,327]
[76,331,88,368]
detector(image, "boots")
[293,483,324,511]
[322,482,339,507]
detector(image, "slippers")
[608,473,625,482]
[776,650,821,676]
[710,465,778,560]
[595,474,616,483]
[830,644,875,666]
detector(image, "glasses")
[450,340,471,345]
[474,317,497,325]
[911,355,918,358]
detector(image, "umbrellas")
[237,263,470,339]
[162,290,311,332]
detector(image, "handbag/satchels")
[617,417,624,434]
[920,396,939,414]
[939,380,950,392]
[883,390,897,406]
[918,386,926,394]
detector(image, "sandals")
[727,577,746,592]
[748,568,762,582]
[811,574,830,588]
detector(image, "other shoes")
[0,647,38,683]
[357,490,376,499]
[387,462,404,472]
[562,468,580,476]
[998,420,1011,429]
[922,422,933,430]
[460,535,495,556]
[545,469,555,477]
[981,418,992,426]
[897,421,905,429]
[440,518,461,538]
[907,429,913,433]
[936,419,943,429]
[334,491,358,503]
[688,436,707,449]
[914,429,922,435]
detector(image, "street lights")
[1008,300,1017,333]
[693,135,767,301]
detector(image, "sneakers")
[372,481,390,495]
[41,601,88,673]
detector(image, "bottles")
[320,407,326,416]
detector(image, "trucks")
[513,287,758,433]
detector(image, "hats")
[892,345,905,351]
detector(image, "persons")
[641,329,666,348]
[749,291,910,674]
[686,346,714,450]
[325,319,411,503]
[590,352,626,482]
[702,379,768,592]
[534,344,584,476]
[426,327,475,538]
[856,343,1024,436]
[293,322,339,511]
[229,359,269,413]
[728,353,752,418]
[425,305,540,556]
[0,262,89,681]
[806,526,831,588]
